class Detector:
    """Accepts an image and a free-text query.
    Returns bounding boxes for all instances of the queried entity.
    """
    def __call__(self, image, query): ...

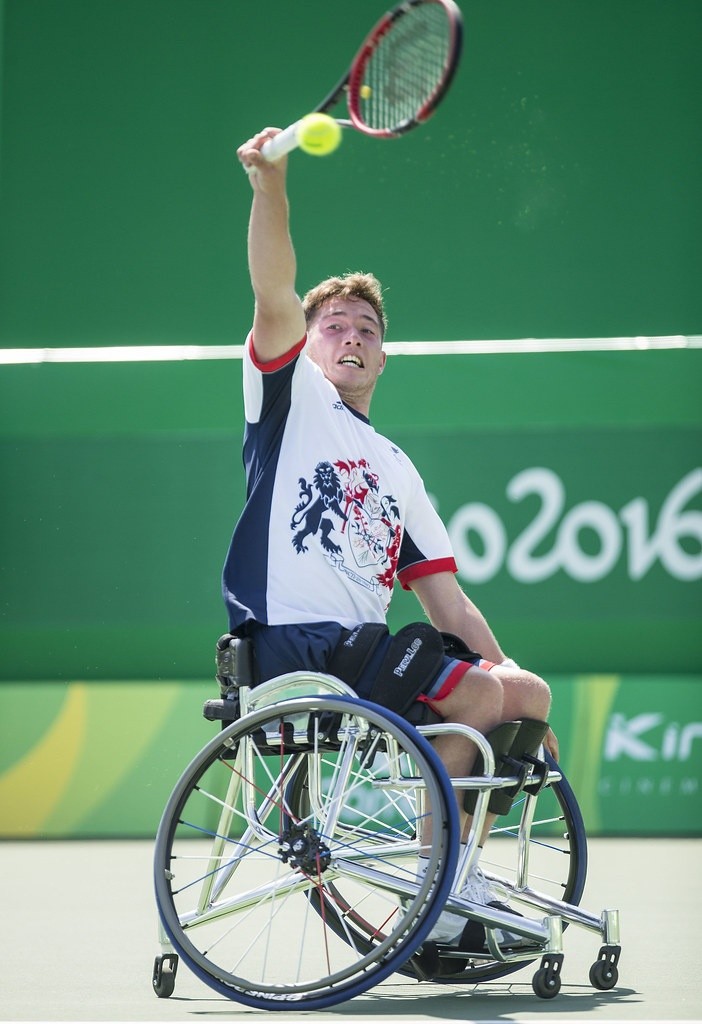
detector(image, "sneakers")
[392,890,523,950]
[457,865,541,947]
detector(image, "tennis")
[295,112,343,155]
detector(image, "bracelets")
[501,657,522,669]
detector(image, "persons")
[223,127,560,956]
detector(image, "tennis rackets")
[241,0,466,173]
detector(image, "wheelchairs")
[150,632,625,1014]
